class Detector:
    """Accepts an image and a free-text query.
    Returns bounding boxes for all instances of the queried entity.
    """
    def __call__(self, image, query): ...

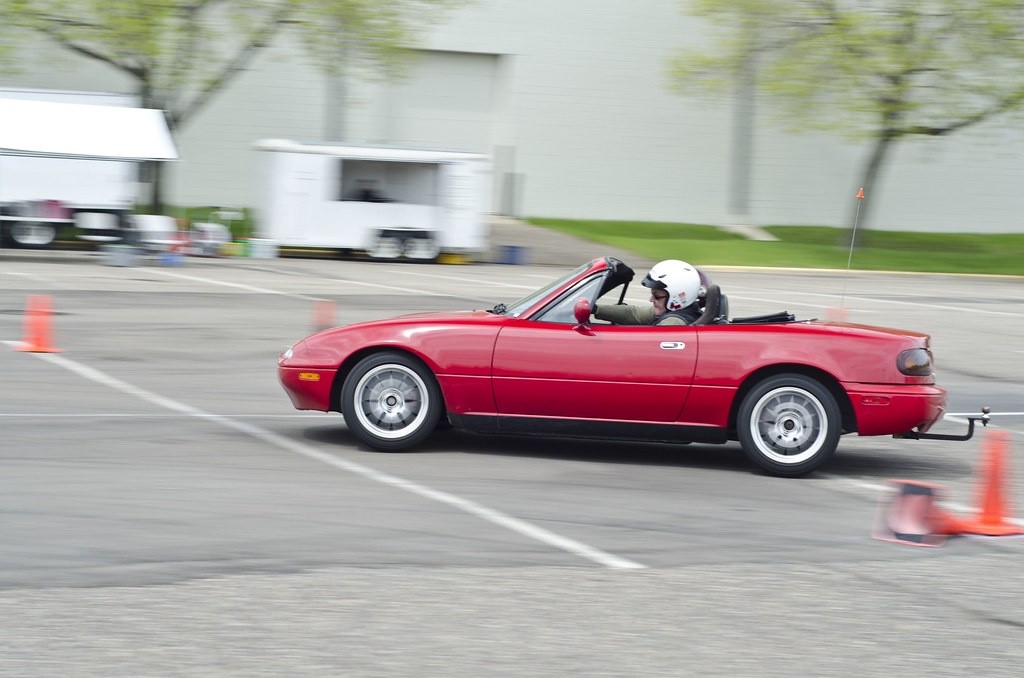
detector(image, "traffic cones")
[10,293,62,354]
[928,426,1024,535]
[872,476,983,549]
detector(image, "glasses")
[651,290,667,300]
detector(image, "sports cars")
[276,255,951,479]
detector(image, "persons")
[341,177,398,203]
[555,259,708,327]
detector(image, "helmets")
[696,269,710,308]
[641,259,701,311]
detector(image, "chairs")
[719,295,729,320]
[690,284,721,325]
